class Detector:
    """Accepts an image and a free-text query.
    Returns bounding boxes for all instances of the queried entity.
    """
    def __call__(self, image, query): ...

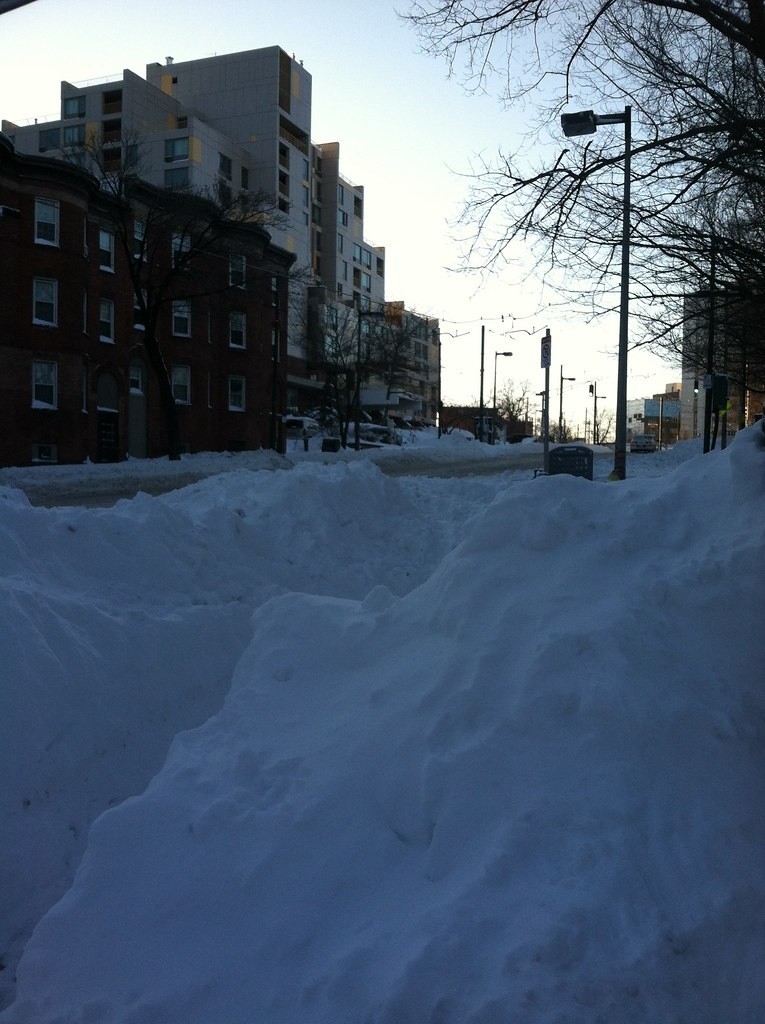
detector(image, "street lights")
[558,375,576,443]
[489,351,513,445]
[560,104,634,482]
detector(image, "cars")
[284,415,332,440]
[345,422,412,451]
